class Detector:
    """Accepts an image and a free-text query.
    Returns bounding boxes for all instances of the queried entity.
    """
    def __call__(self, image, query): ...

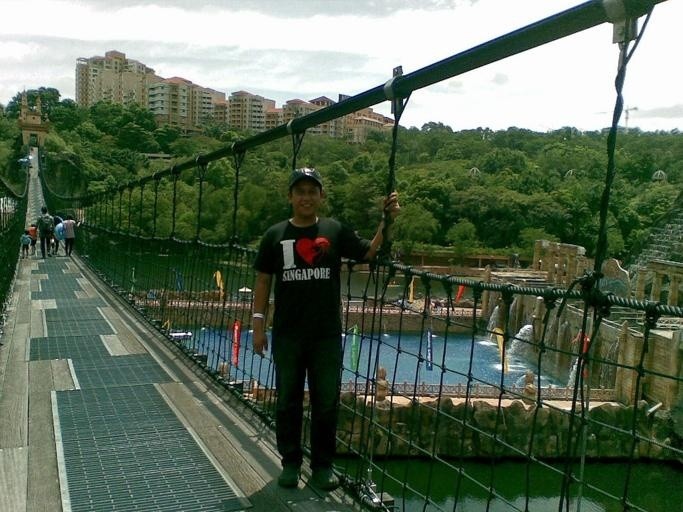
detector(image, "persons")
[33,206,53,259]
[48,212,63,255]
[58,214,80,256]
[250,166,404,490]
[18,229,32,259]
[25,224,37,254]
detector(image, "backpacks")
[39,217,54,233]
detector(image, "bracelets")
[380,215,395,225]
[251,312,264,321]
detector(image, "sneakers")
[48,253,52,257]
[277,466,302,487]
[313,467,340,489]
[53,250,58,254]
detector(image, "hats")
[287,168,323,190]
